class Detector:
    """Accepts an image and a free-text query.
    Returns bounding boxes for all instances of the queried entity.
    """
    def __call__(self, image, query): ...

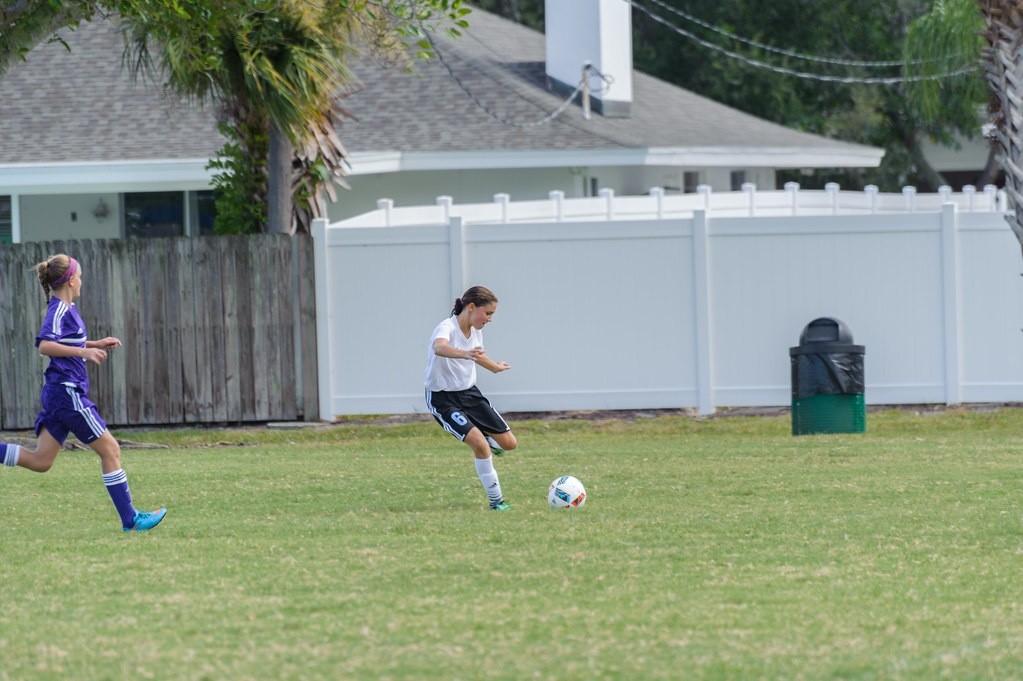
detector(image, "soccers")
[547,475,587,512]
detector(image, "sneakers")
[122,508,167,532]
[486,436,505,457]
[490,501,512,512]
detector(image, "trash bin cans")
[788,316,866,438]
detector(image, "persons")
[0,254,167,532]
[424,286,517,511]
